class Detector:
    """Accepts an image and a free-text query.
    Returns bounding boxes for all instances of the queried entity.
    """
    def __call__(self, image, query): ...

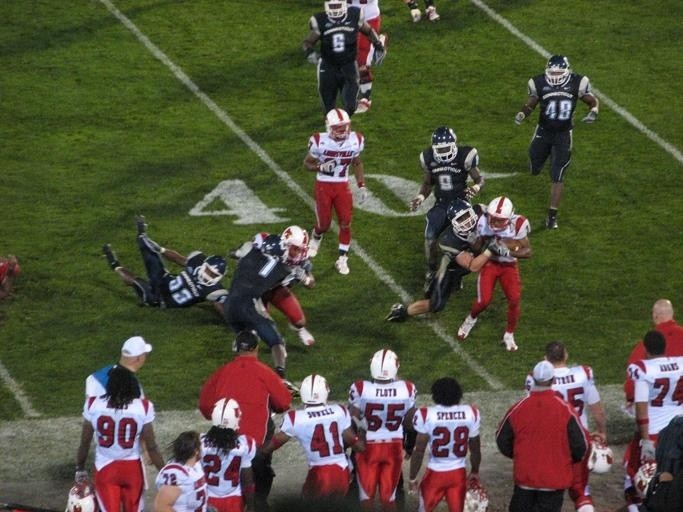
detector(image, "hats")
[120,337,152,359]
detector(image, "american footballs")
[501,239,523,251]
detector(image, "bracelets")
[590,106,598,115]
[415,194,425,203]
[471,183,481,192]
[357,182,366,188]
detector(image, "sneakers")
[136,215,147,232]
[103,243,116,264]
[290,323,315,347]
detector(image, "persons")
[456,193,534,352]
[382,198,499,324]
[302,107,368,274]
[287,1,387,118]
[495,297,682,511]
[403,1,441,23]
[103,215,229,313]
[77,336,481,512]
[408,125,486,284]
[345,0,382,115]
[227,227,314,377]
[512,54,600,233]
